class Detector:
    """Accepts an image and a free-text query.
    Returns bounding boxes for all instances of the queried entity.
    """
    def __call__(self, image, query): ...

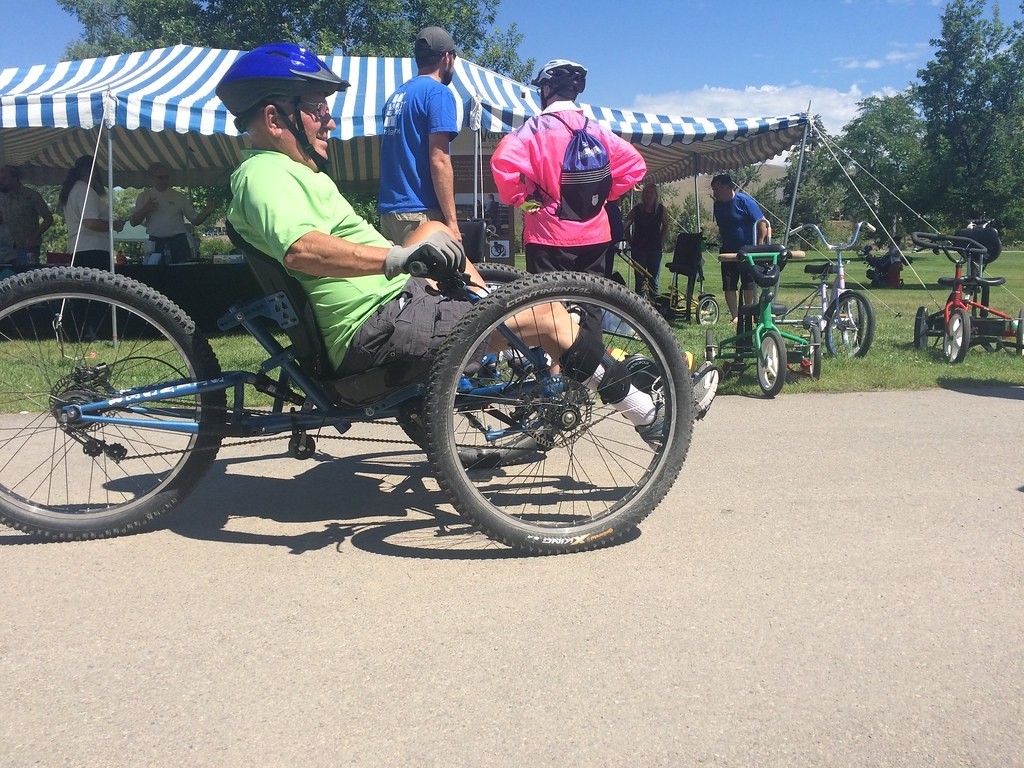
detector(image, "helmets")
[215,42,351,117]
[531,59,587,92]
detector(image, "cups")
[143,240,156,265]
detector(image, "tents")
[0,43,812,348]
[112,220,148,241]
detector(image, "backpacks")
[534,113,614,221]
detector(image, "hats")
[414,26,461,57]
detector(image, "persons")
[491,58,647,376]
[59,155,124,341]
[486,194,498,220]
[376,26,462,248]
[130,162,216,265]
[604,194,623,281]
[215,42,720,451]
[116,251,127,263]
[888,236,901,289]
[711,174,767,324]
[0,167,53,267]
[624,183,669,299]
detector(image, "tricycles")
[706,246,822,395]
[0,261,697,557]
[788,222,877,358]
[909,219,1024,364]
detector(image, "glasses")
[152,175,170,179]
[267,98,328,118]
[536,87,541,96]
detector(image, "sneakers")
[634,361,719,452]
[508,304,583,379]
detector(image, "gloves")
[384,230,467,281]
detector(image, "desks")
[0,265,284,341]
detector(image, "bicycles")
[613,230,719,327]
